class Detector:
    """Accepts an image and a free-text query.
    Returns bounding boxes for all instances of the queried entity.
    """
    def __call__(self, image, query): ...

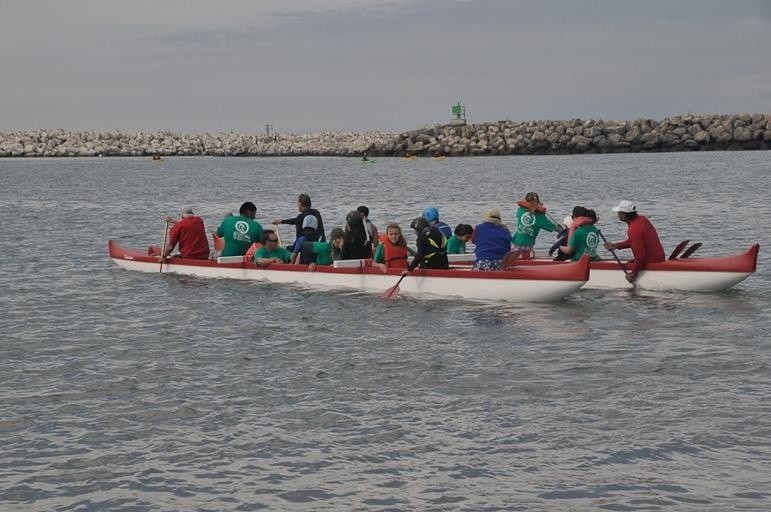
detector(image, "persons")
[431,149,442,157]
[405,152,414,158]
[215,194,376,272]
[153,152,160,160]
[604,201,665,280]
[401,217,449,276]
[470,207,512,271]
[559,206,603,264]
[422,207,452,240]
[510,192,561,258]
[360,152,372,161]
[447,223,473,253]
[372,224,417,270]
[159,207,210,263]
[548,210,575,261]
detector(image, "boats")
[108,237,593,306]
[358,159,376,164]
[401,155,418,161]
[211,230,761,297]
[153,158,166,163]
[431,156,446,160]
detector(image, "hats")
[330,227,344,240]
[484,209,503,225]
[564,216,573,227]
[613,200,638,214]
[179,207,194,217]
[221,212,234,221]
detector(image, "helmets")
[303,215,318,230]
[411,207,440,232]
[346,210,363,226]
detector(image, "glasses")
[267,238,278,242]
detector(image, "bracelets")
[162,255,166,258]
[630,272,636,279]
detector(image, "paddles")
[668,239,702,260]
[502,250,521,268]
[381,275,406,299]
